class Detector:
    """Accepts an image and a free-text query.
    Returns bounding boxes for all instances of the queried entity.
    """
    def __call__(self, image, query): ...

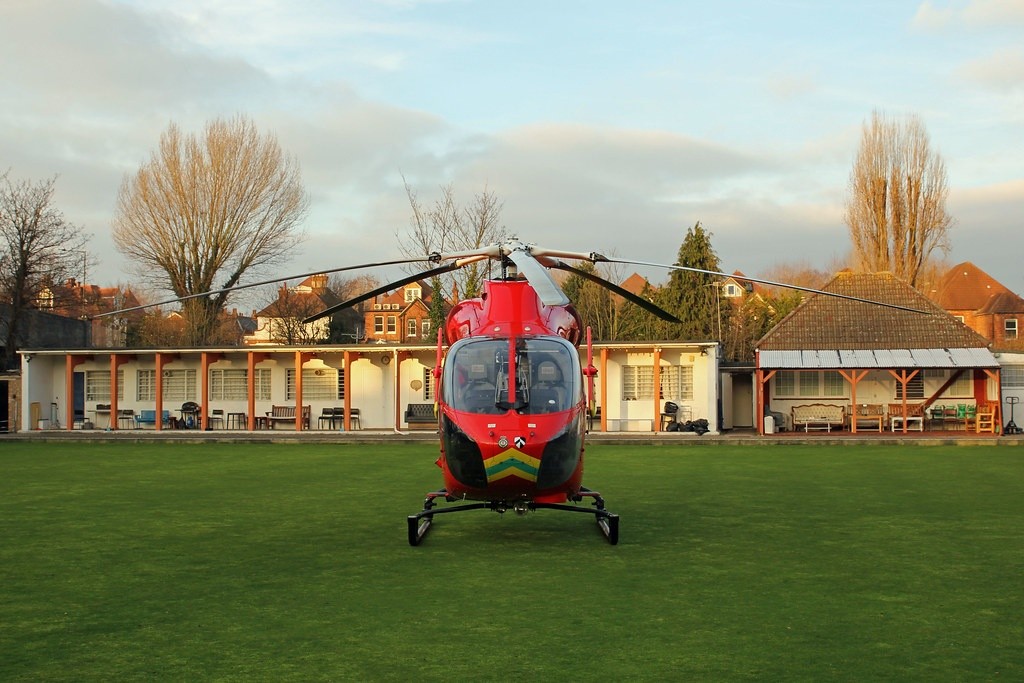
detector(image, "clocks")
[381,356,390,365]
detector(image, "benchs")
[134,410,169,429]
[265,405,311,430]
[847,404,885,431]
[887,403,927,432]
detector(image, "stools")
[197,417,210,429]
[74,417,91,430]
[168,417,177,430]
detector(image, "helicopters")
[91,240,933,545]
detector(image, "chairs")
[330,407,344,430]
[530,362,565,413]
[318,408,333,430]
[976,406,996,434]
[765,405,784,432]
[209,410,223,429]
[350,408,360,430]
[966,404,976,430]
[460,359,499,413]
[588,407,601,430]
[119,410,134,430]
[943,405,957,431]
[928,407,944,432]
[957,404,967,430]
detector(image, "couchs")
[790,403,846,432]
[404,403,439,430]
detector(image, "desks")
[174,409,199,429]
[247,416,267,429]
[849,415,883,432]
[891,417,923,432]
[86,409,111,429]
[660,413,676,430]
[800,419,834,433]
[227,413,246,429]
